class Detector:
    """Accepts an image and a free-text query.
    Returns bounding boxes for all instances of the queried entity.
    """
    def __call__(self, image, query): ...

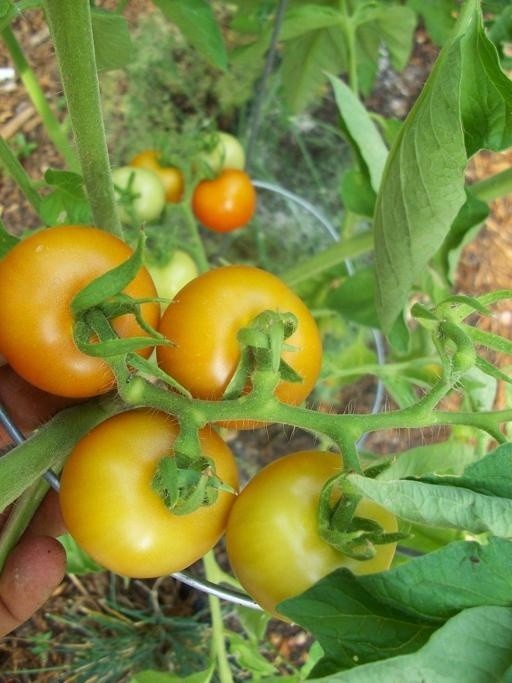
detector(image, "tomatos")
[145,252,199,305]
[190,169,255,232]
[225,447,397,616]
[130,149,186,205]
[0,225,161,398]
[60,410,244,581]
[196,132,246,180]
[113,165,166,229]
[156,265,324,429]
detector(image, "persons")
[0,354,78,640]
